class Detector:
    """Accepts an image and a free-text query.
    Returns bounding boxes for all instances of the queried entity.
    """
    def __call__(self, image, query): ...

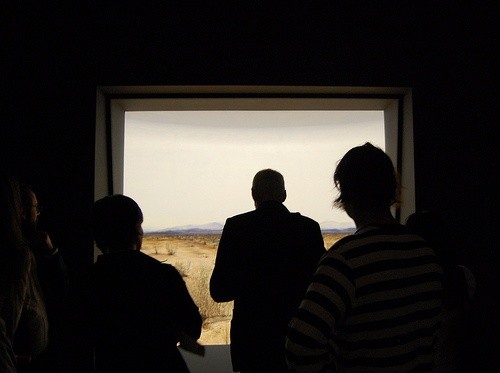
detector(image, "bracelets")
[52,249,58,256]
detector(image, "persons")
[284,143,445,373]
[211,170,326,373]
[0,186,202,373]
